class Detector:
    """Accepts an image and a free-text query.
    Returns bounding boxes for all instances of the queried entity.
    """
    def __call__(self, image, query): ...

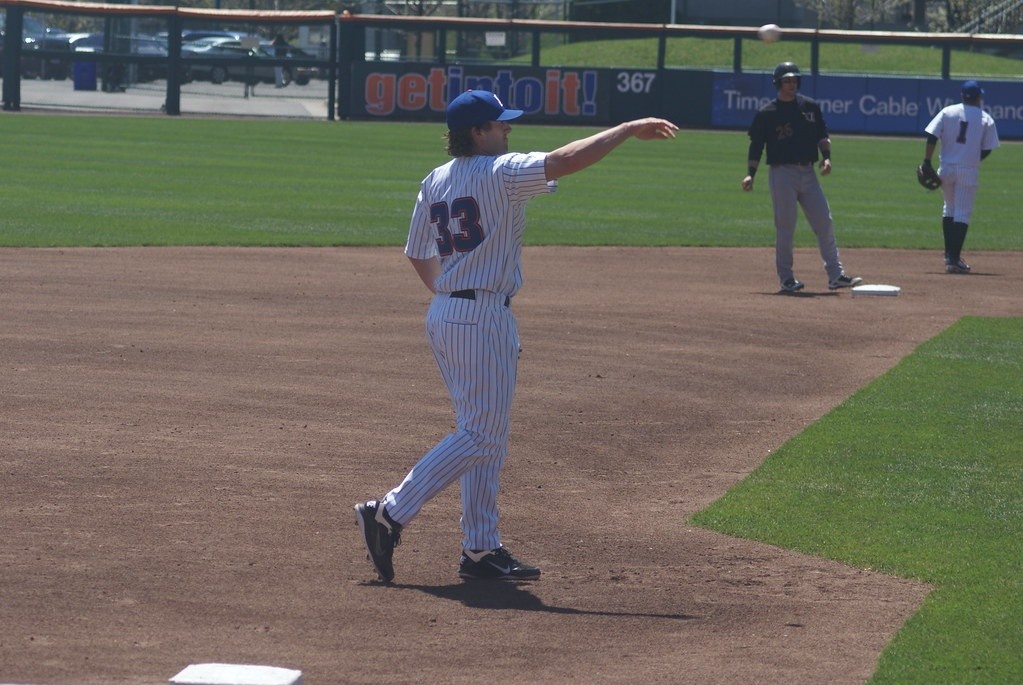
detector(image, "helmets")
[772,61,802,83]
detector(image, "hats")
[446,89,524,130]
[961,80,984,101]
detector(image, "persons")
[742,60,864,292]
[354,88,680,586]
[916,81,1000,274]
[245,46,260,98]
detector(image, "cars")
[181,36,297,88]
[68,30,170,85]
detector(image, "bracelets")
[821,149,832,159]
[746,167,756,178]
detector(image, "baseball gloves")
[918,158,941,190]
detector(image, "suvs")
[0,11,72,80]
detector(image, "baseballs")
[761,23,782,43]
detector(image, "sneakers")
[456,541,541,580]
[828,275,862,288]
[944,251,971,273]
[780,277,804,291]
[355,501,401,581]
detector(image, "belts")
[450,289,510,308]
[789,160,814,166]
